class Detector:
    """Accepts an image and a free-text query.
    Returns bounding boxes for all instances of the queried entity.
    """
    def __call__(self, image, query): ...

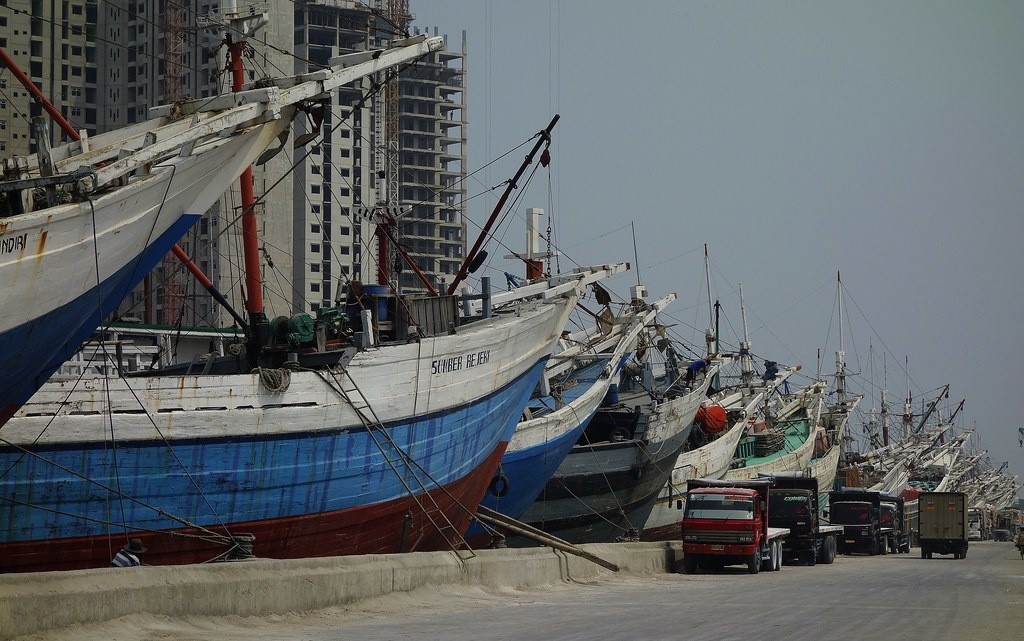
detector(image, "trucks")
[968,509,999,541]
[917,487,970,559]
[998,511,1022,543]
[755,475,844,565]
[681,477,790,574]
[968,505,1024,543]
[881,494,920,554]
[824,491,894,556]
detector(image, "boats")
[0,31,1023,578]
